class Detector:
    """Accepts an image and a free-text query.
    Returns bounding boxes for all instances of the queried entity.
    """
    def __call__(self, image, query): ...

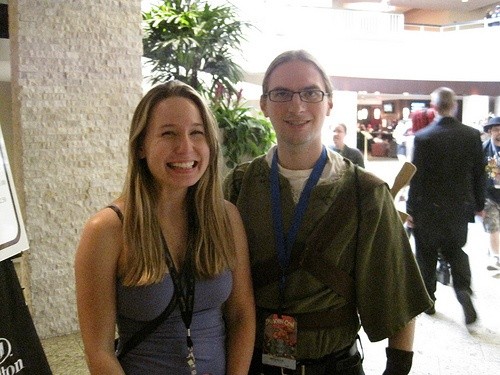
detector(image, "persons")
[482,116,500,278]
[323,121,364,167]
[407,88,484,326]
[75,79,256,375]
[221,50,430,375]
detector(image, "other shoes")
[487,256,500,270]
[437,270,449,284]
[426,298,436,313]
[458,291,476,324]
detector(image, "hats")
[483,117,500,131]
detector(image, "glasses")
[265,89,328,103]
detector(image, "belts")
[254,343,357,375]
